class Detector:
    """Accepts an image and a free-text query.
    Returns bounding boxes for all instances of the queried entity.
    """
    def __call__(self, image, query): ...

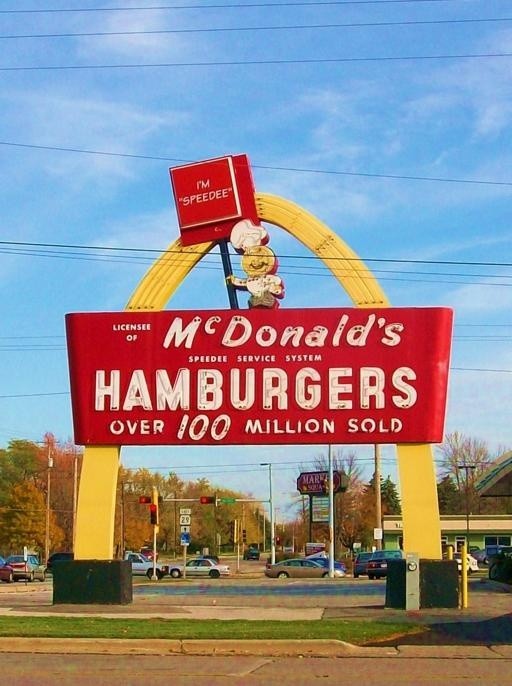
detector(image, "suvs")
[48,552,75,575]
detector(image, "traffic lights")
[139,496,152,504]
[200,496,215,505]
[276,535,280,545]
[150,505,157,524]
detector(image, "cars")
[263,543,512,586]
[7,555,47,582]
[0,555,14,584]
[125,548,229,579]
[243,548,260,561]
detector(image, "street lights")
[260,463,276,565]
[458,466,477,552]
